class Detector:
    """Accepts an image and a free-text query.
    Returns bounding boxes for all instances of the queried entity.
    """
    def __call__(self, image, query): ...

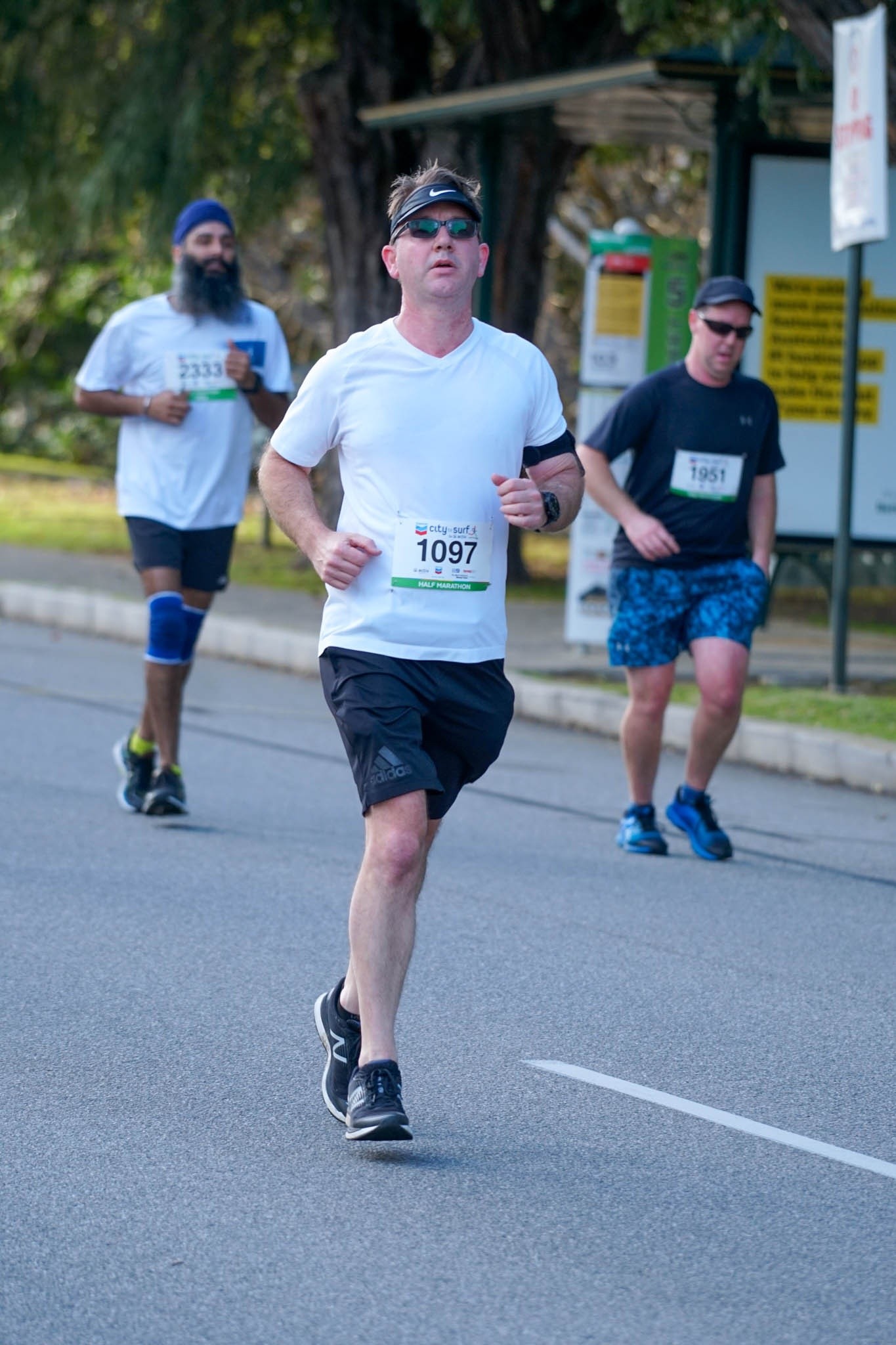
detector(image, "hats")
[171,198,236,246]
[388,183,482,235]
[692,275,763,316]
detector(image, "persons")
[73,198,299,818]
[576,277,790,861]
[258,162,588,1141]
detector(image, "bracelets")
[141,394,153,416]
[238,371,264,396]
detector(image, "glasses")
[697,312,753,339]
[389,217,480,244]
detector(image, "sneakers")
[316,977,361,1121]
[141,766,189,814]
[346,1062,419,1145]
[112,727,157,813]
[667,786,735,861]
[615,805,670,854]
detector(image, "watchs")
[534,490,562,533]
